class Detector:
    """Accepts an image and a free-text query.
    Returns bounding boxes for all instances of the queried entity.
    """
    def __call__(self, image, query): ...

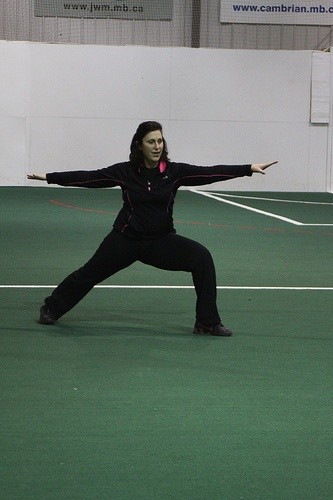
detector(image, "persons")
[26,120,278,336]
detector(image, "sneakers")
[39,306,56,323]
[194,323,233,336]
